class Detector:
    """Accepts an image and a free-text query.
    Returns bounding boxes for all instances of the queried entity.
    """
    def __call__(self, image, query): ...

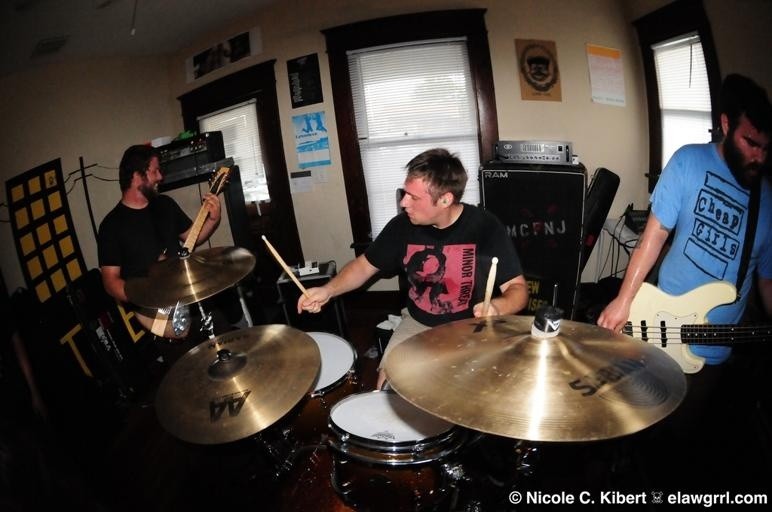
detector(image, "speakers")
[277,262,349,339]
[478,159,588,321]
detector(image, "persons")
[97,145,242,341]
[297,149,529,389]
[598,74,772,373]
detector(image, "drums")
[328,388,471,511]
[288,331,358,445]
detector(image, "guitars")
[620,281,772,374]
[134,166,230,343]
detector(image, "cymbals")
[124,246,257,310]
[153,324,321,445]
[383,315,688,443]
